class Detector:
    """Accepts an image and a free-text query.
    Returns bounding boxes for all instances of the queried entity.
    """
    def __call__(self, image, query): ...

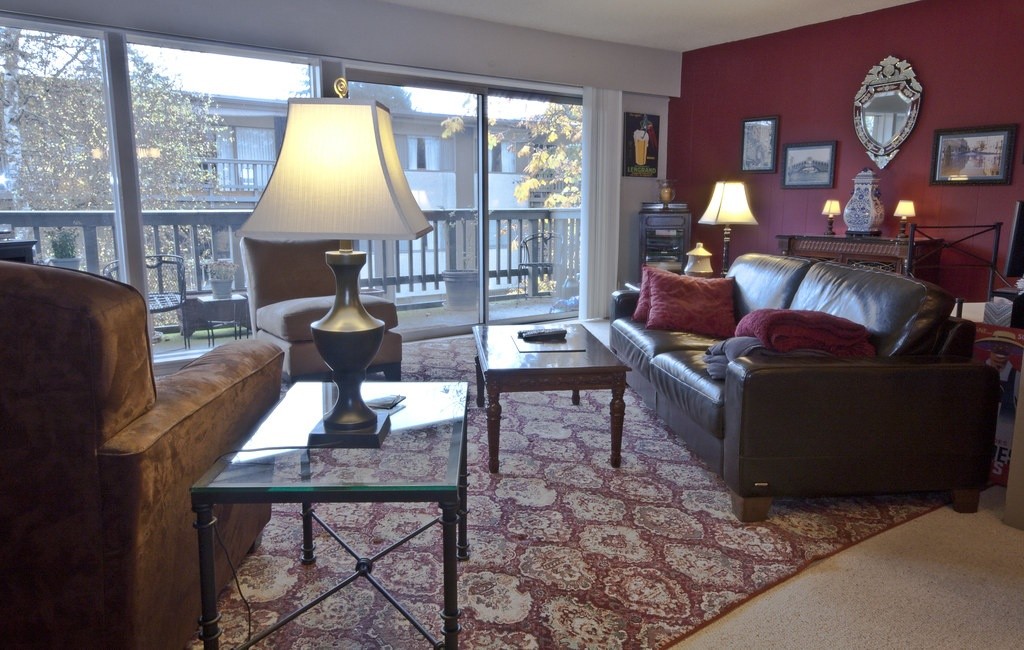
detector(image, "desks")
[196,294,249,347]
[0,237,38,264]
[777,234,943,283]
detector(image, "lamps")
[238,97,433,450]
[697,181,759,278]
[821,200,842,235]
[893,200,916,238]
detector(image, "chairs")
[102,254,190,351]
[516,232,565,306]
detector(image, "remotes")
[518,328,566,339]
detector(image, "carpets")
[192,337,1002,650]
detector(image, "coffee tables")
[471,322,632,474]
[190,378,469,650]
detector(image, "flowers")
[439,205,492,271]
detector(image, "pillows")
[632,264,684,321]
[646,266,737,339]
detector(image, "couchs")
[0,258,285,650]
[607,252,1000,523]
[241,235,403,385]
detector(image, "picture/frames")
[780,140,838,189]
[738,115,780,174]
[929,122,1018,191]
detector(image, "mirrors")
[853,57,923,168]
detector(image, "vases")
[442,270,480,311]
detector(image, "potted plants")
[47,228,81,272]
[207,260,235,300]
